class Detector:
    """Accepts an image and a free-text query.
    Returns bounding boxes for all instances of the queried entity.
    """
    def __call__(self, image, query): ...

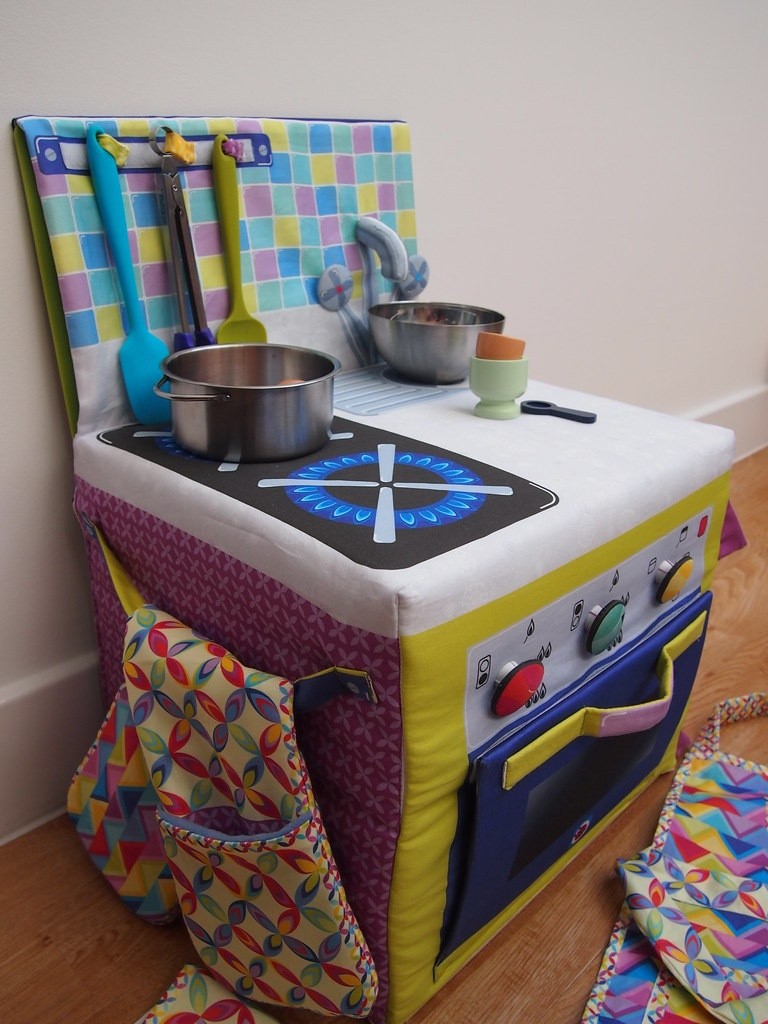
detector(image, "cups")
[467,330,530,420]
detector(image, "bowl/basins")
[366,300,505,385]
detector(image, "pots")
[154,343,343,464]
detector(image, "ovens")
[390,438,732,997]
